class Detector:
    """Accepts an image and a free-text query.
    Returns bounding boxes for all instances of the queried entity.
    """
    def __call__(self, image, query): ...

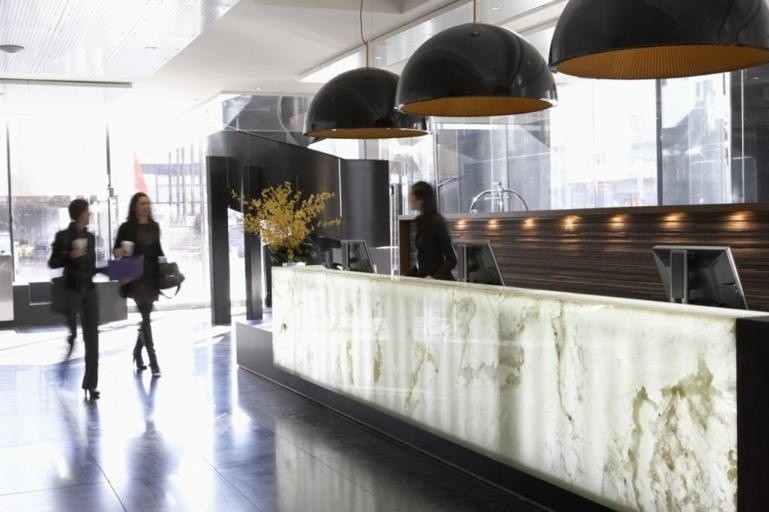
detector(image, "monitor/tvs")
[652,246,749,310]
[452,240,505,285]
[340,240,375,273]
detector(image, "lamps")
[300,37,430,139]
[549,1,769,81]
[395,23,558,120]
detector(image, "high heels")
[133,349,147,369]
[82,382,100,399]
[149,360,161,377]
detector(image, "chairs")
[12,280,55,329]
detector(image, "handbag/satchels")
[51,277,66,314]
[159,262,185,299]
[107,254,143,281]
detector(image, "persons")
[111,192,164,377]
[408,181,458,281]
[47,200,100,399]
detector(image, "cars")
[0,232,50,274]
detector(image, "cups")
[121,240,134,257]
